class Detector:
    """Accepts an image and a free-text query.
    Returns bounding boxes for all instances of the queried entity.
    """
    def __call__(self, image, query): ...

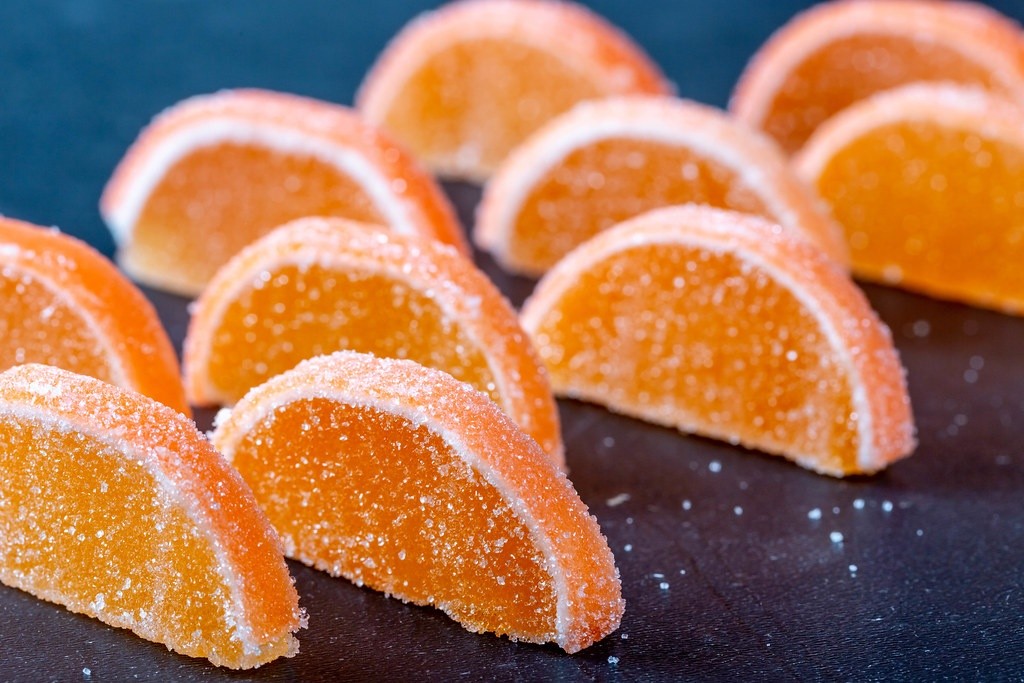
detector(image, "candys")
[0,0,1024,670]
